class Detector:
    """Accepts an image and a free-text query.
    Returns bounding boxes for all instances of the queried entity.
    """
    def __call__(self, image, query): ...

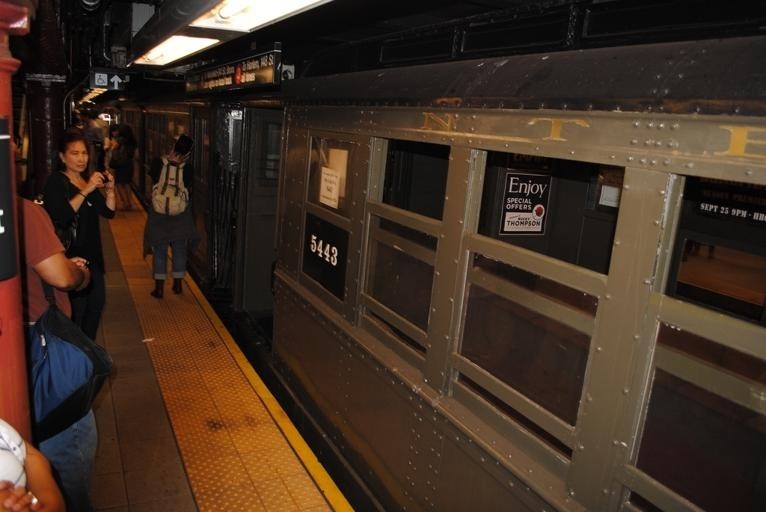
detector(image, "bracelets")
[75,192,87,199]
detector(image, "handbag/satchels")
[27,305,112,442]
[56,214,79,251]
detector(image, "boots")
[173,279,181,292]
[151,280,163,297]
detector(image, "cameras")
[102,174,109,183]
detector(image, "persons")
[41,129,117,344]
[17,197,99,511]
[145,134,201,299]
[110,124,137,212]
[72,105,118,183]
[0,418,65,512]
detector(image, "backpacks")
[152,156,190,216]
